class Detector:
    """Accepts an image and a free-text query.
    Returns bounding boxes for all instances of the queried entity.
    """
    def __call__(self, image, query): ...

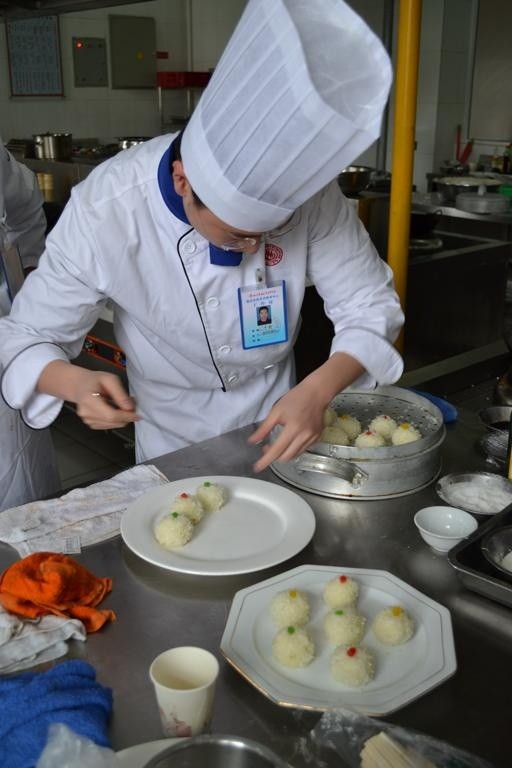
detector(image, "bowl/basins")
[482,522,512,578]
[143,731,293,767]
[338,165,374,193]
[433,178,503,201]
[411,504,476,551]
[477,405,512,462]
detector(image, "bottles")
[491,145,512,175]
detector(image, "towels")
[1,610,89,672]
[0,460,173,558]
[1,547,117,632]
[0,657,116,767]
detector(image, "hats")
[177,53,395,234]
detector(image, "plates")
[435,470,512,517]
[115,736,190,767]
[227,662,324,742]
[120,540,313,603]
[405,388,459,425]
[122,475,316,579]
[220,561,462,717]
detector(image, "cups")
[411,209,443,239]
[500,184,512,196]
[146,645,218,737]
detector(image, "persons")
[256,304,271,325]
[0,125,408,474]
[0,139,63,512]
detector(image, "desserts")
[157,512,193,547]
[321,409,421,448]
[325,606,367,643]
[274,628,316,667]
[198,476,225,511]
[330,644,375,684]
[322,573,360,607]
[375,604,413,646]
[271,589,308,627]
[171,491,204,528]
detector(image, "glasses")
[222,207,304,254]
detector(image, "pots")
[118,136,154,151]
[31,132,73,161]
